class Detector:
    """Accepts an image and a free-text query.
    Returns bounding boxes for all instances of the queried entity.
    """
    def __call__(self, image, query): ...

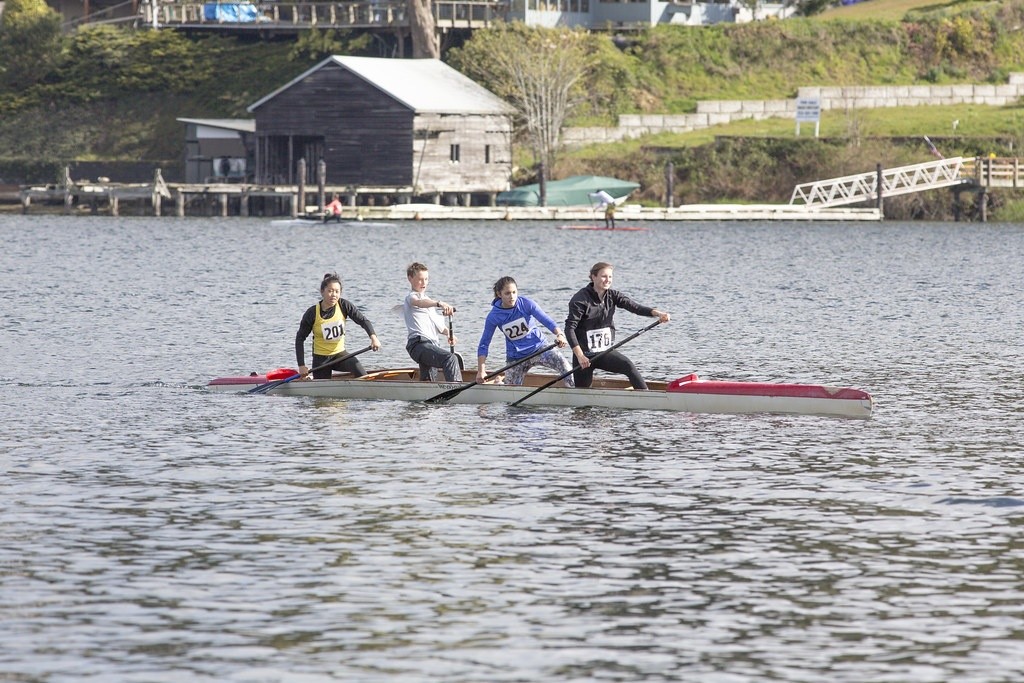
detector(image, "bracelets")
[557,334,565,338]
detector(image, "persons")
[295,274,381,379]
[323,194,342,224]
[587,190,616,228]
[476,277,575,388]
[564,262,670,390]
[405,263,462,381]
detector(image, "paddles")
[441,307,464,370]
[588,195,598,227]
[510,318,662,407]
[248,345,373,395]
[423,339,560,403]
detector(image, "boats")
[205,368,874,419]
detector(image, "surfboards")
[555,224,647,232]
